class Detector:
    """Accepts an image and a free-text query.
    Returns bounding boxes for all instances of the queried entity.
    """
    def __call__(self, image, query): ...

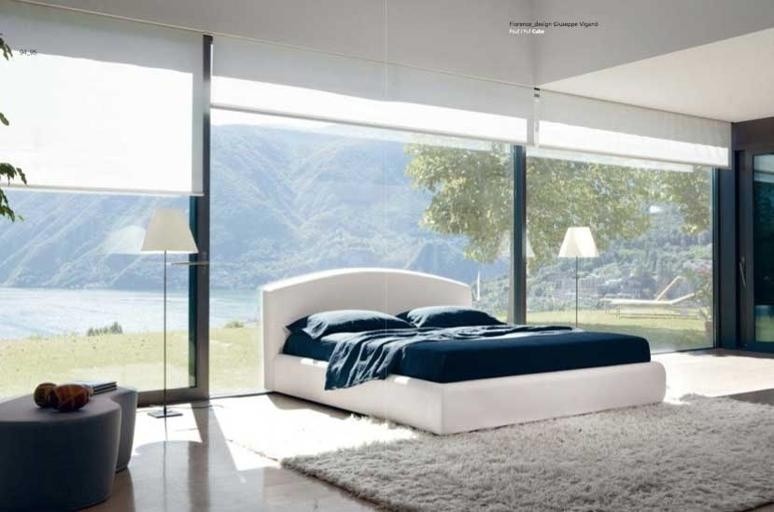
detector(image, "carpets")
[229,391,774,512]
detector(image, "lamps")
[141,209,199,418]
[558,226,600,329]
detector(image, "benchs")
[0,385,138,512]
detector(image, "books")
[83,380,117,395]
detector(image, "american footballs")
[47,385,93,412]
[35,383,56,408]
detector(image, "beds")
[262,268,667,436]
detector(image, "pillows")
[286,305,507,342]
